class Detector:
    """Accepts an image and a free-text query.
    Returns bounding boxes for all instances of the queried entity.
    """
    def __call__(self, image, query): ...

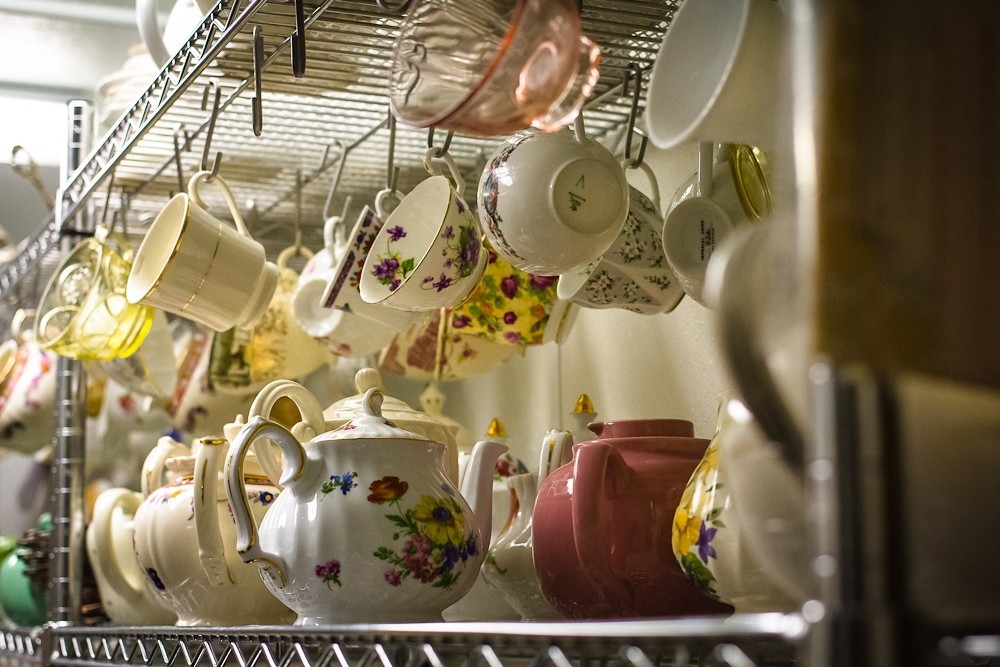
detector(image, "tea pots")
[0,213,1000,633]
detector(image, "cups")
[0,1,794,548]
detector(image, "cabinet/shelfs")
[0,1,854,667]
